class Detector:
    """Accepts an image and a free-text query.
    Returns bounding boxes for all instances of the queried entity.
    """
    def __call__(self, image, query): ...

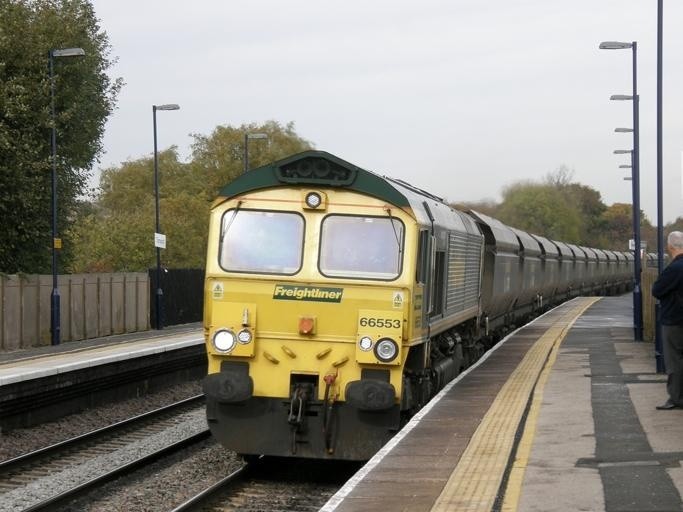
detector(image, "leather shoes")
[656,399,683,409]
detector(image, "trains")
[194,149,675,464]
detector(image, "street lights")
[149,104,179,329]
[597,40,645,343]
[240,131,268,174]
[43,46,86,350]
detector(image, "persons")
[651,230,683,410]
